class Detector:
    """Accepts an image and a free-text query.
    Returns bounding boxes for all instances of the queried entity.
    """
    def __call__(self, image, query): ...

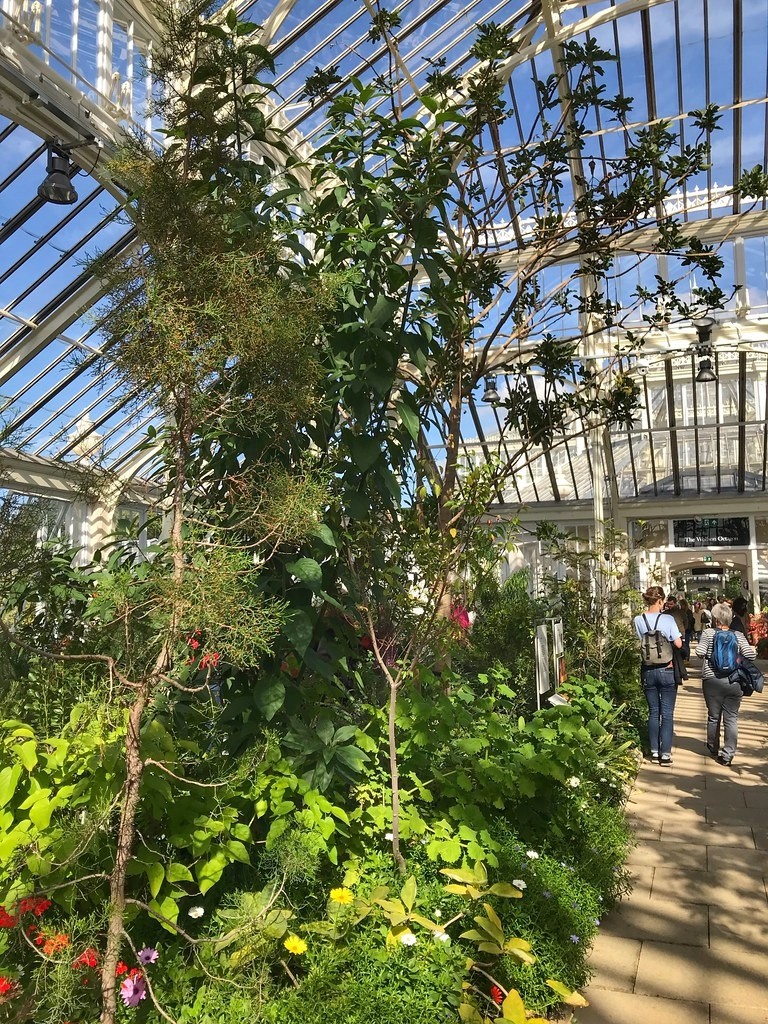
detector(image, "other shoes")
[683,660,690,667]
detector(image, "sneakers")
[707,743,718,756]
[660,758,673,766]
[651,755,661,764]
[717,756,731,766]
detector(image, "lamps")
[37,136,105,205]
[481,371,501,402]
[692,316,718,382]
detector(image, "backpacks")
[705,628,742,673]
[639,613,674,665]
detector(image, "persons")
[633,585,684,766]
[695,602,756,765]
[452,596,478,630]
[662,595,755,687]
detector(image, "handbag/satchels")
[701,612,710,623]
